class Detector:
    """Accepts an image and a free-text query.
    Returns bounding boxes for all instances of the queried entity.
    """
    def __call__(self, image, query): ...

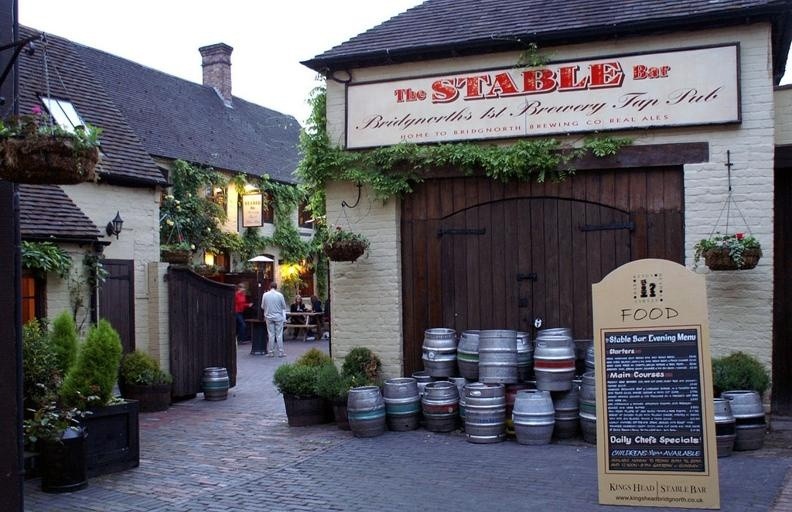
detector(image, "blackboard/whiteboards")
[600,324,709,476]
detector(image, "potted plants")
[21,310,176,493]
[324,227,371,262]
[272,347,381,430]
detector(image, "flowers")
[692,232,761,272]
[1,104,104,176]
[160,231,190,250]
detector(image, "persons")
[290,294,323,341]
[261,281,287,358]
[231,283,253,345]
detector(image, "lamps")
[106,211,123,239]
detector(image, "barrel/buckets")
[121,380,173,413]
[348,326,596,447]
[201,366,230,401]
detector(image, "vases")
[2,136,99,186]
[703,247,762,270]
[160,250,190,264]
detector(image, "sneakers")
[267,352,274,358]
[278,351,287,358]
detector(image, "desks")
[285,312,324,342]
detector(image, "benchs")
[284,320,325,341]
